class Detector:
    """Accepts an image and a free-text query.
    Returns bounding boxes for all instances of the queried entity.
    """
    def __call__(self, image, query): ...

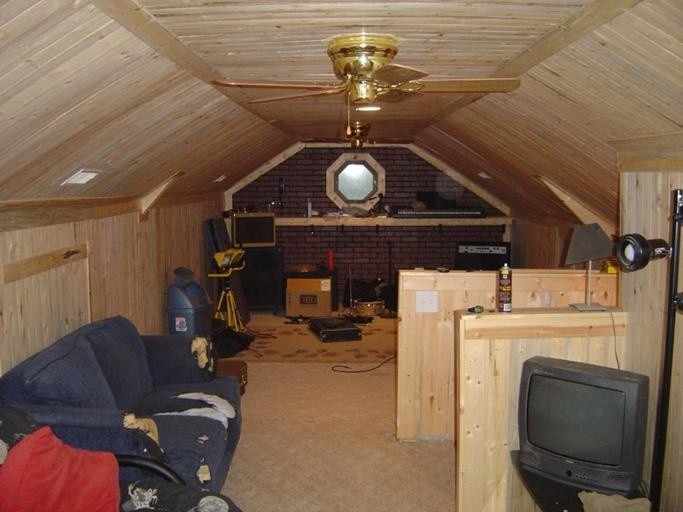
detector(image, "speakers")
[238,248,282,311]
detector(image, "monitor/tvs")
[516,356,649,497]
[233,212,277,248]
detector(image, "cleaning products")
[499,263,512,312]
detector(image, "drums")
[352,299,387,316]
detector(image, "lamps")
[563,224,617,312]
[615,191,683,512]
[347,82,382,111]
[347,122,371,151]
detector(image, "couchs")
[0,316,241,494]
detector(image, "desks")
[510,450,652,512]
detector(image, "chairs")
[0,405,241,512]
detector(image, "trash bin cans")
[166,267,213,339]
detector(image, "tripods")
[207,277,245,332]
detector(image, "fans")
[211,33,521,105]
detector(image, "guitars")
[222,200,281,219]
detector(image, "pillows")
[0,426,121,512]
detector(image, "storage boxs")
[286,274,333,320]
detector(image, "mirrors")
[334,160,378,203]
